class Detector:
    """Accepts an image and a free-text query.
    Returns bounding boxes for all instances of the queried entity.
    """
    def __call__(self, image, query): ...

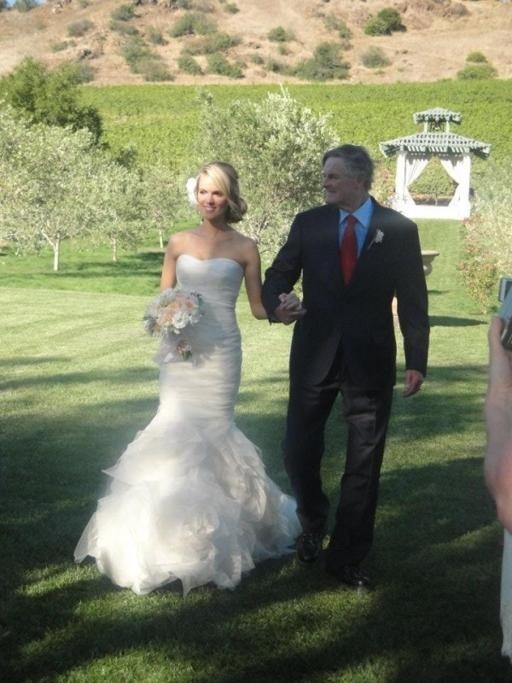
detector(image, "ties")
[340,214,359,287]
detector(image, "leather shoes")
[329,541,372,587]
[295,519,330,566]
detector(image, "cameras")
[497,278,512,348]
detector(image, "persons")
[480,314,511,667]
[74,160,308,601]
[259,141,431,588]
